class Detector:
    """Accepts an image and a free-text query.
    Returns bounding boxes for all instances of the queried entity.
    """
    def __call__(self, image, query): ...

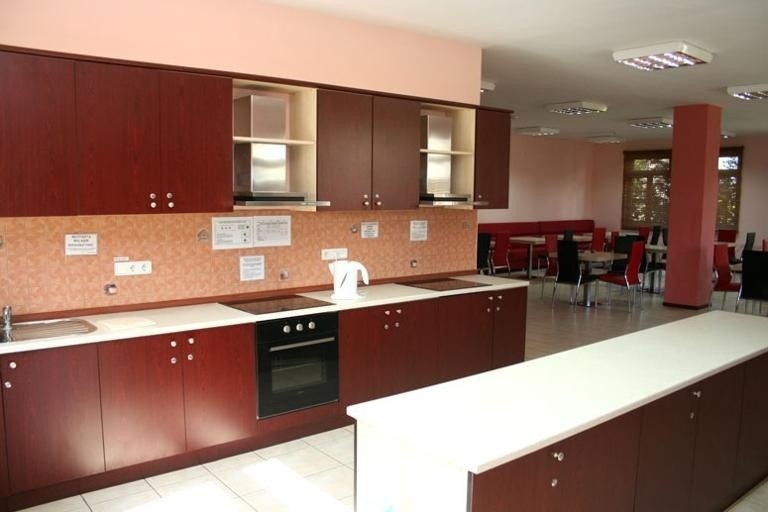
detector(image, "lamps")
[515,41,767,143]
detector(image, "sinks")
[0,329,12,343]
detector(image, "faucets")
[2,305,12,330]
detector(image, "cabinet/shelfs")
[233,134,314,211]
[469,351,768,511]
[476,109,511,209]
[315,89,420,210]
[436,287,526,384]
[1,344,105,495]
[98,324,256,472]
[336,300,436,406]
[420,147,472,206]
[75,61,232,215]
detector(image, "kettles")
[328,259,369,300]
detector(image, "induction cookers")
[393,276,493,292]
[215,293,337,337]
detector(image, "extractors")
[419,113,489,207]
[232,93,331,207]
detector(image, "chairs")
[479,223,768,310]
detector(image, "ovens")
[255,338,338,422]
